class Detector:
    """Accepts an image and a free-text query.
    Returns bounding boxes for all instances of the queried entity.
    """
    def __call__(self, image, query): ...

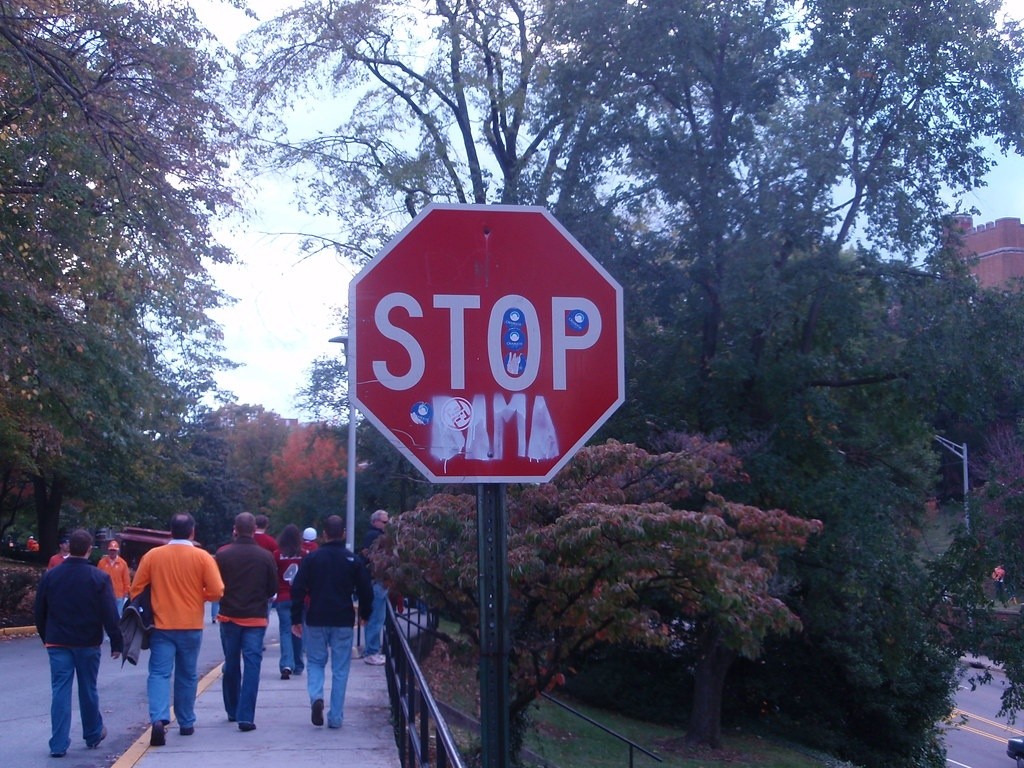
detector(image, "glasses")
[379,519,389,526]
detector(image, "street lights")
[328,336,354,559]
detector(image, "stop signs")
[347,202,624,482]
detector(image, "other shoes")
[280,667,291,679]
[238,722,256,730]
[310,697,326,726]
[148,721,168,745]
[363,654,385,665]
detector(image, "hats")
[303,527,318,540]
[106,540,120,551]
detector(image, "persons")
[291,516,374,728]
[97,540,130,643]
[216,514,277,730]
[5,535,11,544]
[34,530,125,757]
[212,510,389,680]
[130,514,224,745]
[48,536,69,570]
[28,536,39,551]
[991,565,1005,602]
